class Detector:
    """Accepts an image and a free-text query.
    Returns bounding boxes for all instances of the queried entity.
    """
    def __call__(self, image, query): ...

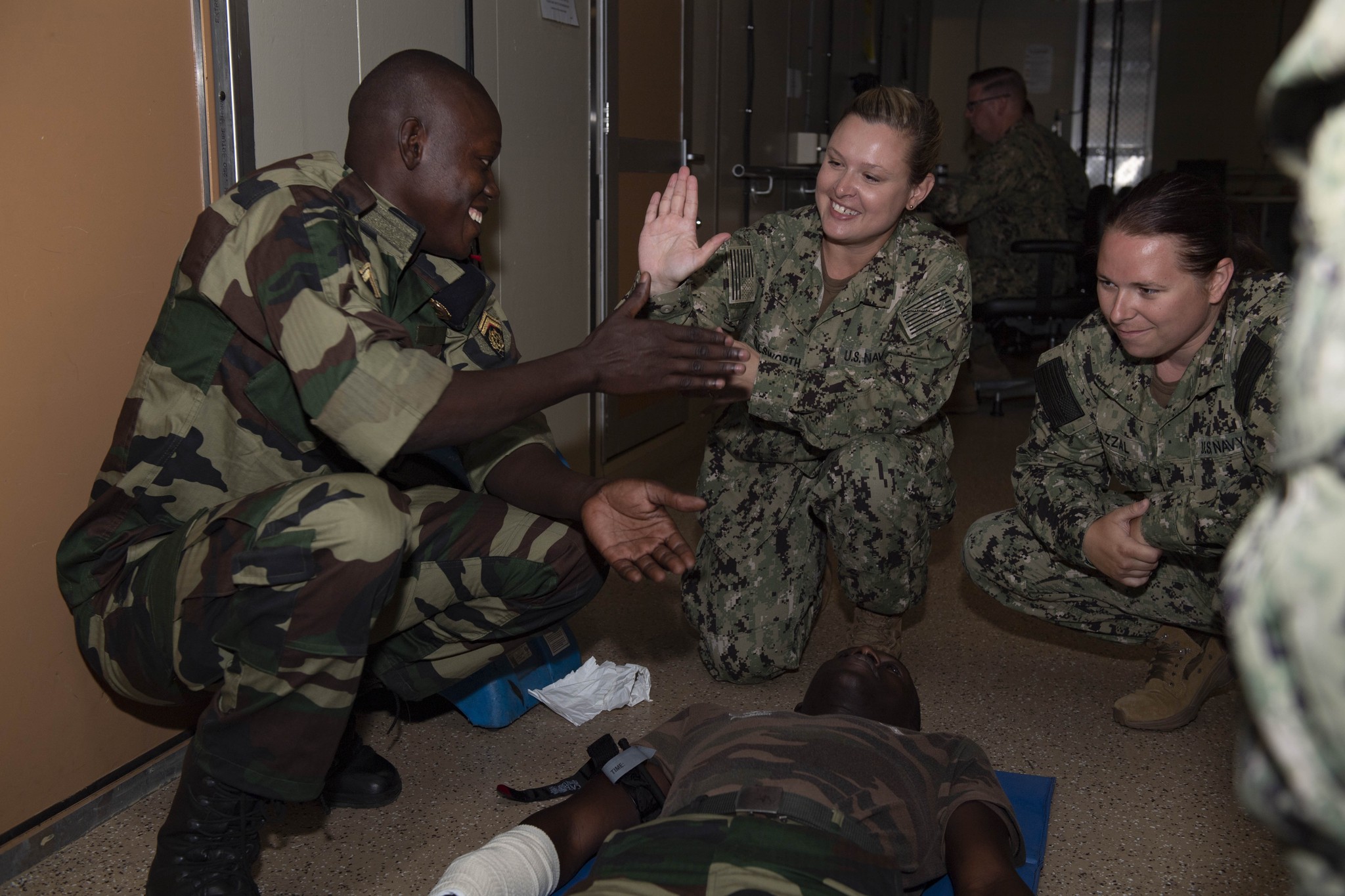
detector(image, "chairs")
[973,167,1165,417]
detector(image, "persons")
[929,67,1091,416]
[962,169,1298,730]
[55,49,752,896]
[1221,0,1345,896]
[428,645,1036,896]
[617,85,972,686]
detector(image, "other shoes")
[955,343,1008,413]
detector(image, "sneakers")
[847,608,903,661]
[1114,623,1232,731]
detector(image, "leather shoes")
[146,761,262,896]
[318,744,402,810]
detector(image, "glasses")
[968,95,1010,113]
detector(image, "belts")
[670,784,883,856]
